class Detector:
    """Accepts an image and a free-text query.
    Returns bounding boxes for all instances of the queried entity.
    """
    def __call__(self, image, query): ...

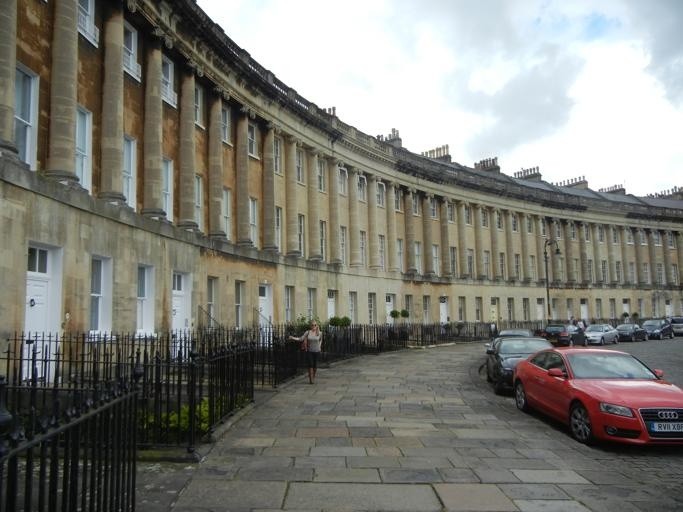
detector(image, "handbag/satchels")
[301,337,308,352]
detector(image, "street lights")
[543,237,565,328]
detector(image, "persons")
[564,314,597,331]
[288,320,322,384]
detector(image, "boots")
[309,368,316,384]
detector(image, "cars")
[483,315,683,397]
[512,344,683,450]
